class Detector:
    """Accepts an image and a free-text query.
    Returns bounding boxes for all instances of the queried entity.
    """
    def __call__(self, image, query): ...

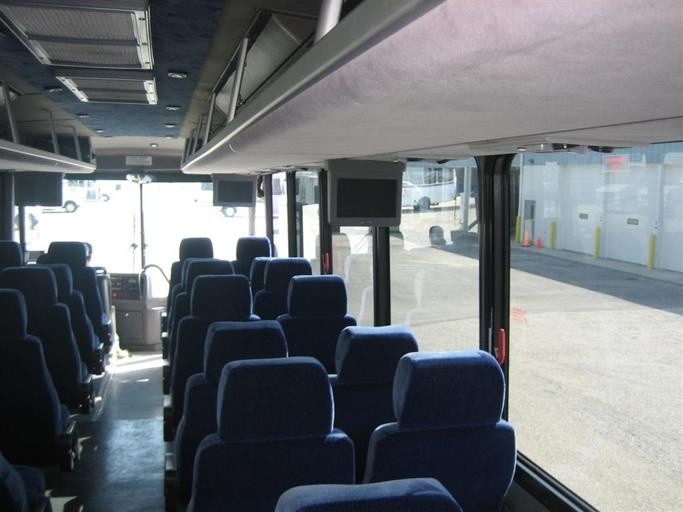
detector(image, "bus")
[398,159,460,214]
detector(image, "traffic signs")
[602,154,629,173]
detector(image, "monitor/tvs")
[328,159,403,227]
[213,174,256,206]
[14,171,63,206]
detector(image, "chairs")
[325,325,419,435]
[167,238,214,314]
[253,257,312,320]
[186,356,356,512]
[167,258,195,332]
[0,287,87,471]
[361,350,517,512]
[231,237,271,274]
[49,264,106,378]
[250,256,271,298]
[0,240,23,269]
[173,260,235,322]
[275,477,462,511]
[276,274,358,374]
[48,241,114,352]
[1,266,97,417]
[172,320,290,479]
[172,274,262,432]
[0,455,53,512]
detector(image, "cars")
[39,181,84,216]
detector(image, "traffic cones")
[536,237,541,248]
[522,230,531,247]
